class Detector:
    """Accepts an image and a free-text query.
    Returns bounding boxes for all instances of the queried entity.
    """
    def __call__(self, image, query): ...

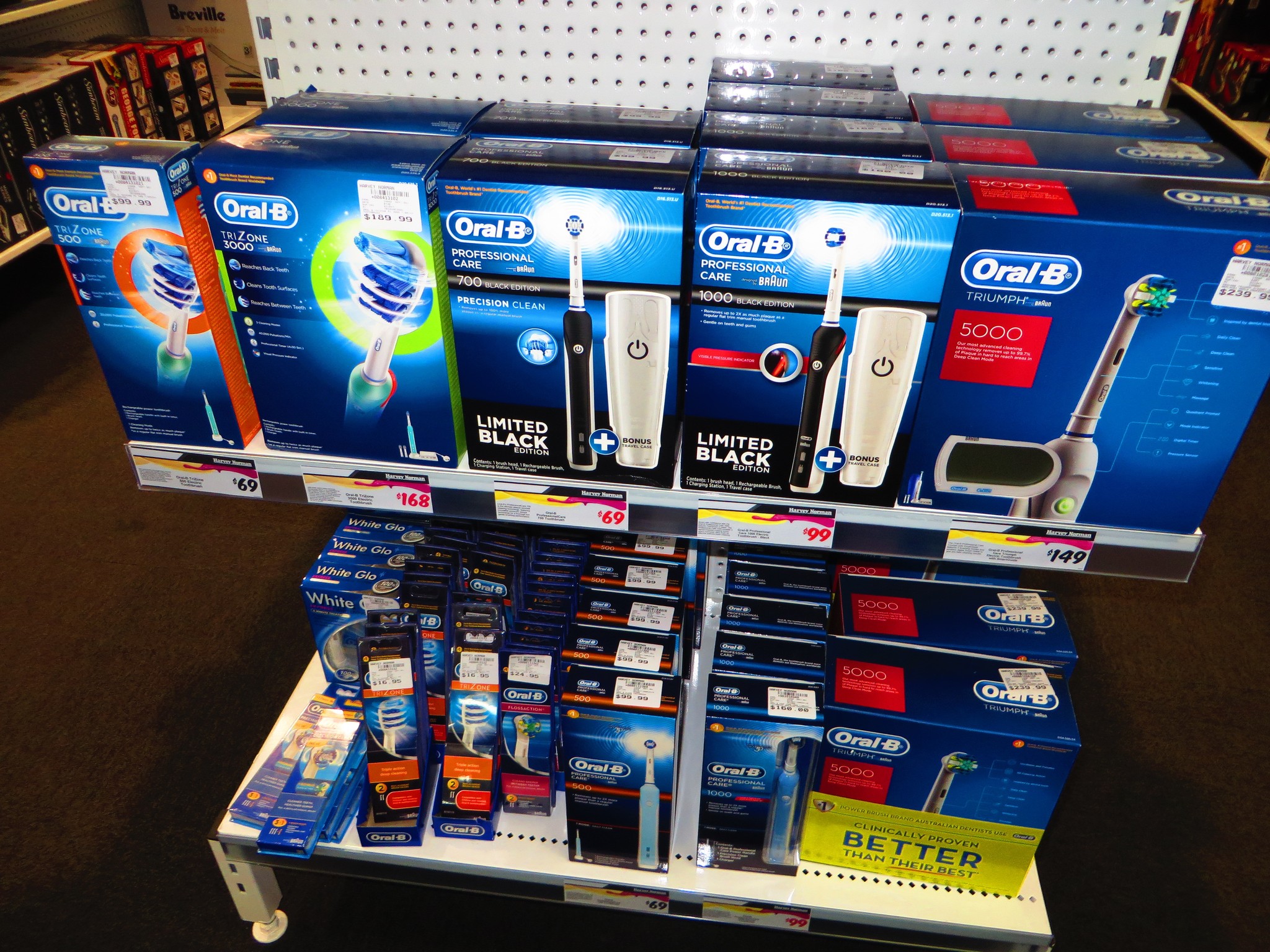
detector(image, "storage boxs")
[6,25,1270,893]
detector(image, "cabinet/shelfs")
[129,429,1203,952]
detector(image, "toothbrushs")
[763,733,806,865]
[635,736,663,871]
[280,726,313,764]
[512,712,543,773]
[421,639,438,675]
[302,744,338,784]
[557,209,603,472]
[786,219,851,496]
[456,694,491,754]
[920,748,979,817]
[1006,271,1180,528]
[377,696,415,761]
[334,227,428,434]
[135,234,205,399]
